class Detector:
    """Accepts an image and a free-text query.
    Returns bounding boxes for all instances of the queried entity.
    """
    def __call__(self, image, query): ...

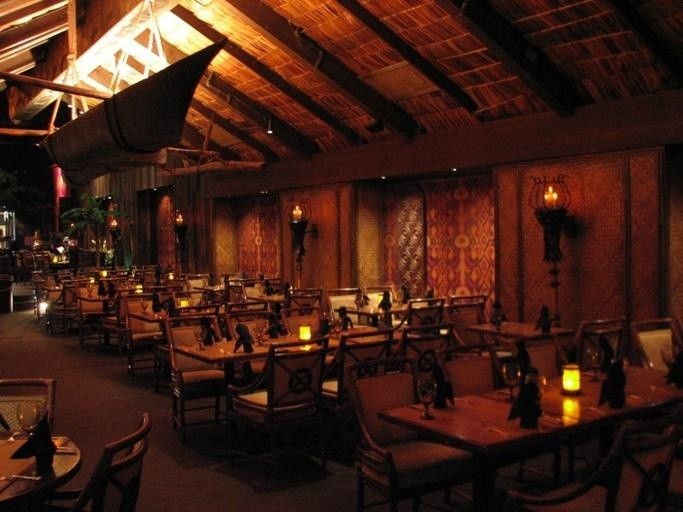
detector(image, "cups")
[561,397,579,427]
[321,312,342,341]
[611,359,629,375]
[17,402,41,435]
[562,364,581,392]
[525,375,546,399]
[298,325,311,341]
[200,295,207,306]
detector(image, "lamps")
[171,210,189,275]
[280,198,318,291]
[527,174,577,326]
[266,118,273,134]
[108,218,120,249]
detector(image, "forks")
[0,475,41,480]
[6,432,18,442]
[219,348,232,355]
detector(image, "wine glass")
[502,363,521,401]
[417,377,437,419]
[254,319,268,346]
[140,301,148,315]
[583,345,603,382]
[87,284,92,298]
[659,344,679,377]
[193,325,207,353]
